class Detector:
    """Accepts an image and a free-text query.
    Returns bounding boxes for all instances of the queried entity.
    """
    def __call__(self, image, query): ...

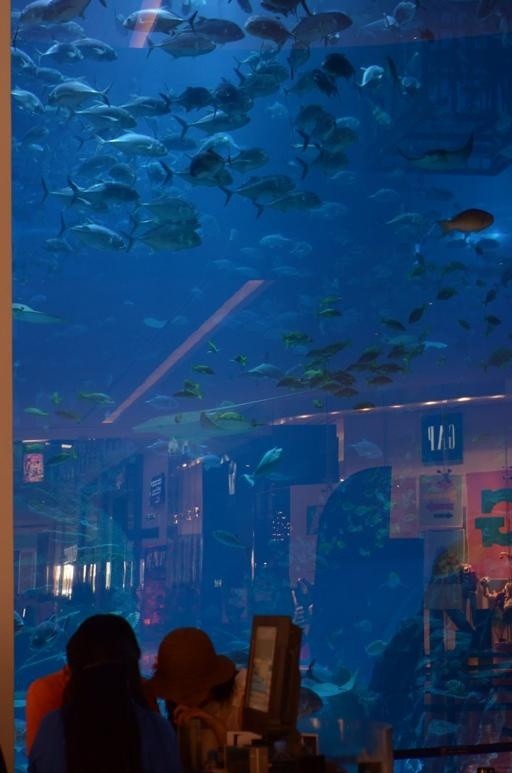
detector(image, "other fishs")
[13,2,511,773]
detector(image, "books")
[240,613,303,735]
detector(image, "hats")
[63,613,141,697]
[423,539,468,612]
[147,625,237,710]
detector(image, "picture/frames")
[389,467,512,593]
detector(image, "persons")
[143,625,249,773]
[25,665,161,758]
[30,613,181,772]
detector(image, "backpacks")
[372,624,424,689]
